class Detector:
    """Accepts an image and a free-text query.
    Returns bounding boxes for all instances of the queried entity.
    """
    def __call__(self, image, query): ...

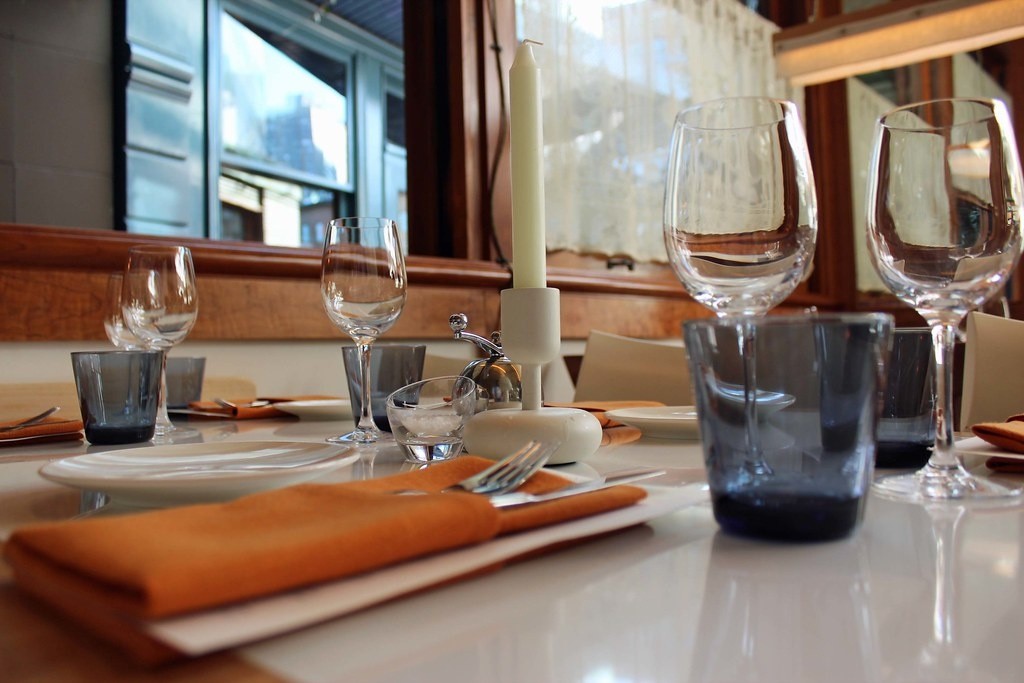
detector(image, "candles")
[508,38,548,288]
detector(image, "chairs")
[958,310,1024,432]
[574,329,692,404]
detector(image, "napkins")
[0,414,86,440]
[192,394,297,420]
[0,462,665,671]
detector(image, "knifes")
[489,468,666,508]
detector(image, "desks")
[1,421,1024,681]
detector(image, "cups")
[165,356,206,421]
[385,375,476,464]
[682,311,895,543]
[70,349,165,446]
[814,313,952,470]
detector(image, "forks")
[382,438,562,496]
[0,406,68,432]
[214,397,350,411]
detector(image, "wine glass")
[102,267,172,423]
[323,216,408,448]
[864,98,1024,510]
[662,95,818,496]
[120,245,199,445]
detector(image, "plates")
[37,440,360,502]
[602,405,703,439]
[143,476,710,657]
